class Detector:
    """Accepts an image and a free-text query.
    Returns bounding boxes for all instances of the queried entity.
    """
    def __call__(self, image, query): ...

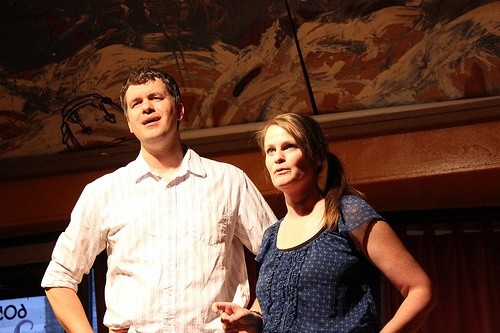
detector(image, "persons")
[210,113,442,333]
[40,66,280,333]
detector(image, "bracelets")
[248,310,263,318]
